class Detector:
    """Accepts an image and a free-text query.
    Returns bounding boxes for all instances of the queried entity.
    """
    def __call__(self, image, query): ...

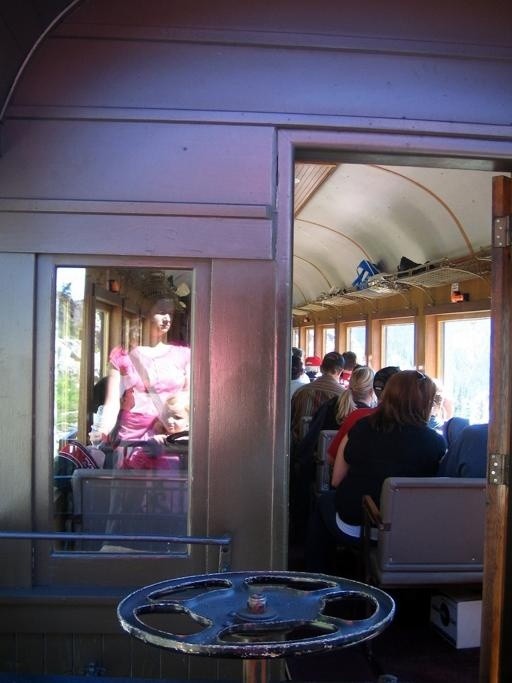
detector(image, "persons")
[91,372,162,454]
[118,390,191,472]
[89,284,191,470]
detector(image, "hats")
[373,367,400,391]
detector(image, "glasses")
[416,371,427,380]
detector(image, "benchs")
[297,414,490,587]
[70,439,187,555]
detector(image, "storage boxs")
[429,591,483,651]
